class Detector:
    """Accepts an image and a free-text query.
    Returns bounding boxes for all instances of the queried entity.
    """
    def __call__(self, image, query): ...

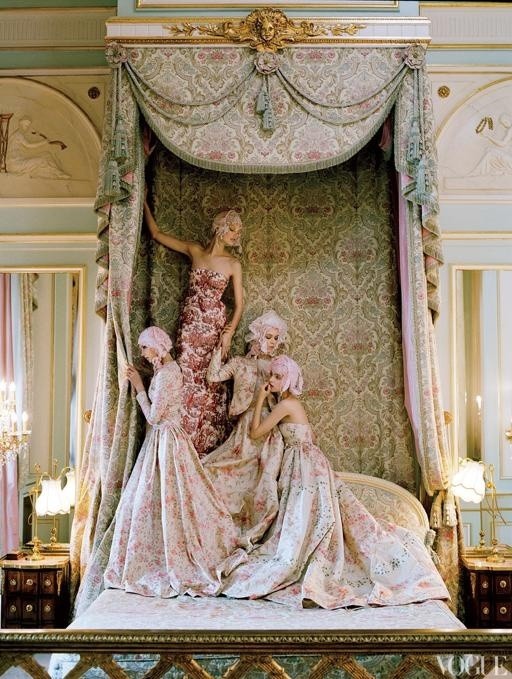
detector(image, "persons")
[106,325,234,598]
[207,307,289,556]
[143,186,245,459]
[250,352,338,608]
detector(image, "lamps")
[1,381,32,466]
[29,472,70,559]
[444,456,508,563]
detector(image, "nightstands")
[459,542,512,630]
[0,554,70,629]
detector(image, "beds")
[46,468,496,678]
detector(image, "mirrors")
[449,266,512,478]
[1,265,85,495]
